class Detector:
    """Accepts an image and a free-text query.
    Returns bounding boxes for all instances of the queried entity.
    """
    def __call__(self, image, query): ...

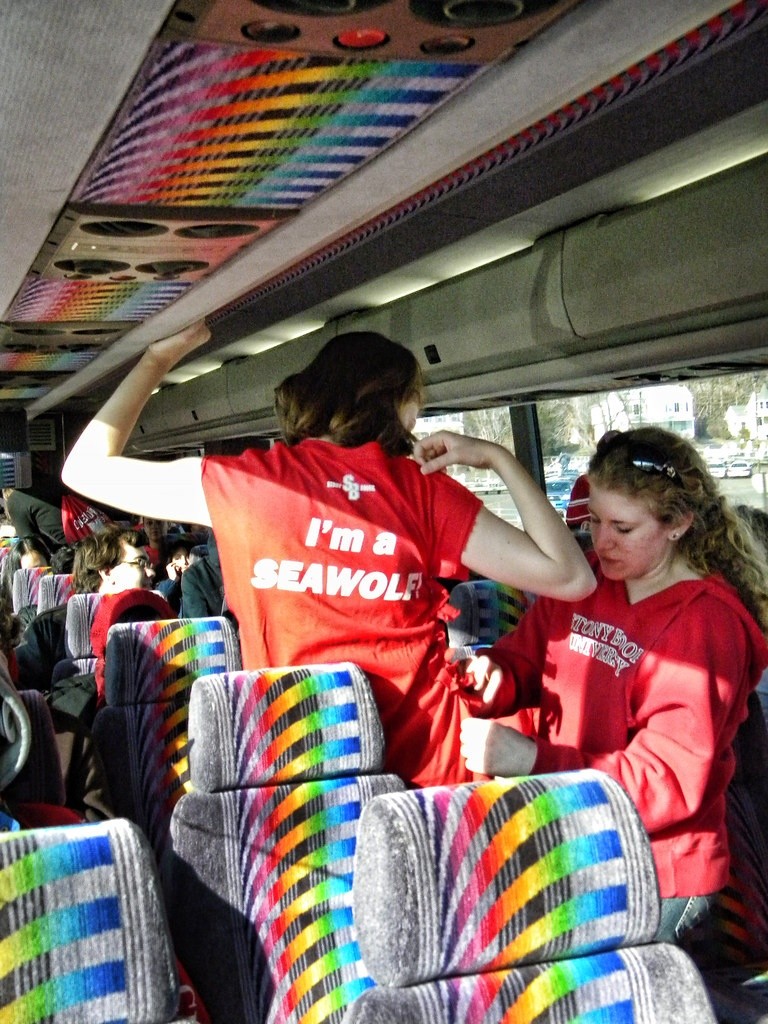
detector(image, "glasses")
[117,558,155,570]
[597,430,685,490]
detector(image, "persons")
[59,317,598,787]
[0,486,768,976]
[459,427,768,941]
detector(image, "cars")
[727,460,751,479]
[706,461,726,480]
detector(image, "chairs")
[88,615,245,858]
[37,575,72,613]
[448,578,535,652]
[54,591,170,682]
[343,767,722,1024]
[0,814,182,1024]
[13,566,53,613]
[0,547,12,585]
[162,660,408,1024]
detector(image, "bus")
[543,473,578,511]
